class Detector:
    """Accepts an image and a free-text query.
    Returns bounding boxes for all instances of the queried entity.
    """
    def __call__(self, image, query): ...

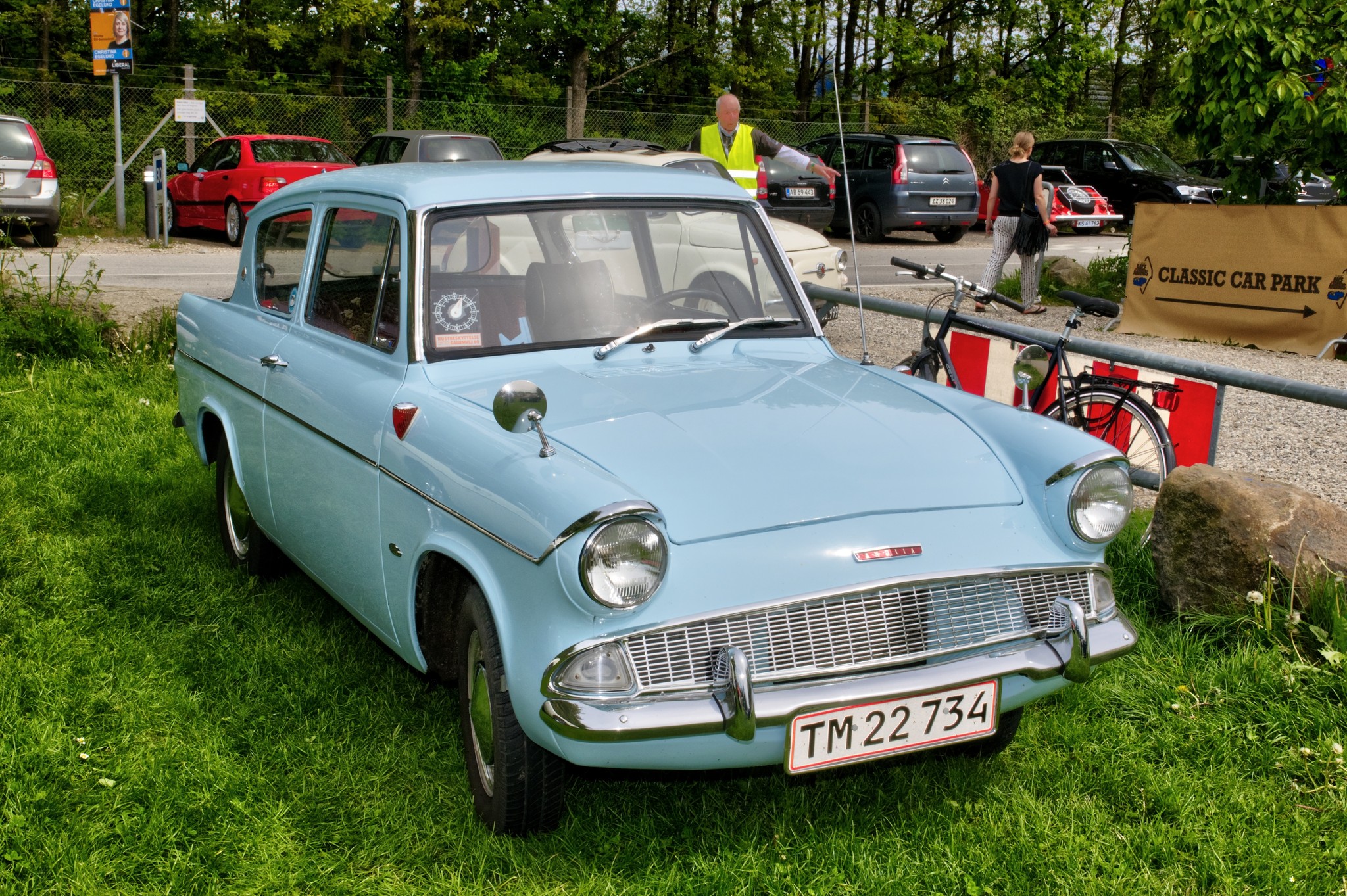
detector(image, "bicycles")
[885,257,1177,555]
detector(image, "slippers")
[975,307,985,312]
[1025,305,1047,314]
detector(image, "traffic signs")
[1119,199,1347,360]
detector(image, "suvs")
[681,129,982,244]
[1026,138,1247,228]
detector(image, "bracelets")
[1043,219,1050,226]
[808,162,816,173]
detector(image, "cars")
[347,130,504,244]
[163,134,378,248]
[444,138,851,337]
[171,162,1140,835]
[1178,156,1340,206]
[978,166,1123,235]
[0,114,61,245]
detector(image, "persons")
[974,132,1058,314]
[687,94,841,202]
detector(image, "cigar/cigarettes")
[1056,234,1057,236]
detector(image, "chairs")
[525,260,618,344]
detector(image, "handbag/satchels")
[1014,205,1050,256]
